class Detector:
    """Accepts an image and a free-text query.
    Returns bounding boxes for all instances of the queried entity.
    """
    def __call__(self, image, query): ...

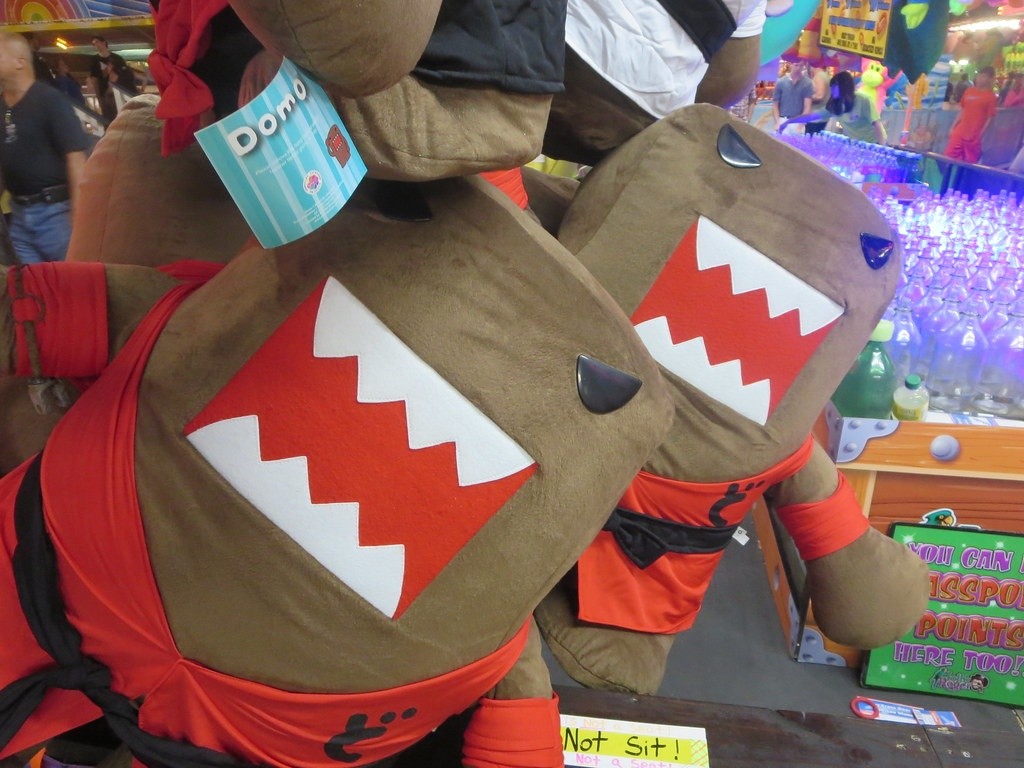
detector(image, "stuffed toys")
[854,54,903,118]
[0,94,931,768]
[146,0,767,182]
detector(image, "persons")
[52,61,87,111]
[772,62,815,131]
[0,31,88,263]
[21,33,57,87]
[944,67,997,165]
[90,37,140,132]
[826,71,888,145]
[945,71,1024,108]
